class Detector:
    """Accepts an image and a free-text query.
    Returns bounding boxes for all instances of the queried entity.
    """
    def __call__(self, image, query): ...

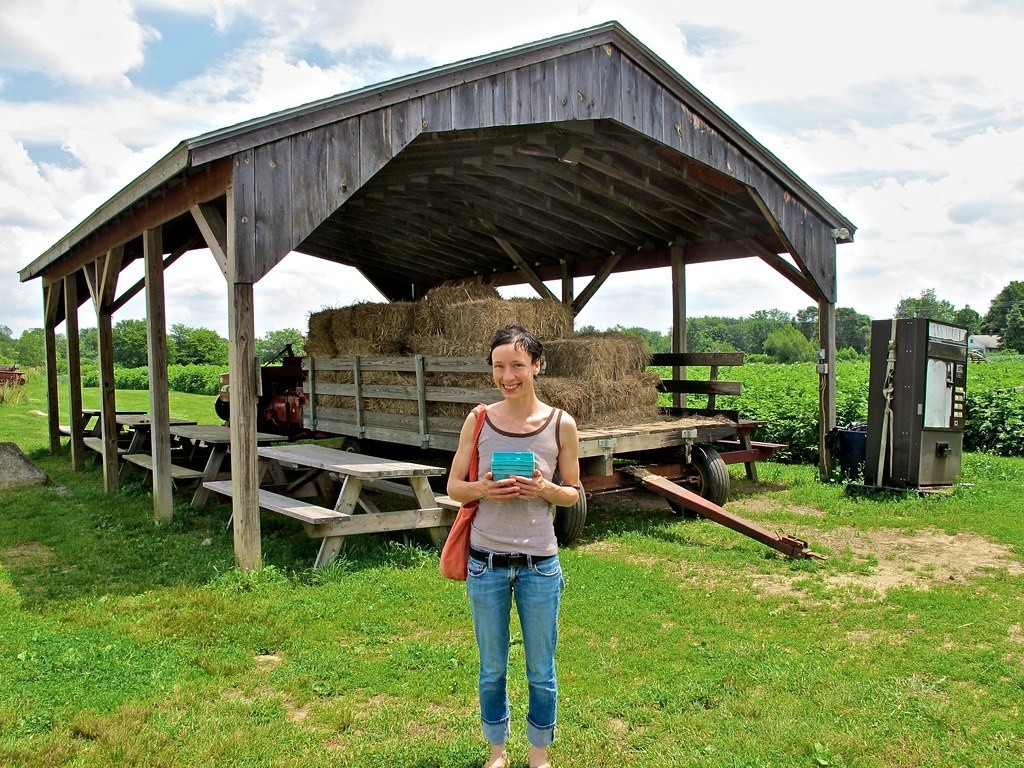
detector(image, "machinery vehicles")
[219,357,731,544]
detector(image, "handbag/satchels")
[439,400,487,581]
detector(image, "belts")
[468,547,558,566]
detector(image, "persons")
[440,327,580,768]
